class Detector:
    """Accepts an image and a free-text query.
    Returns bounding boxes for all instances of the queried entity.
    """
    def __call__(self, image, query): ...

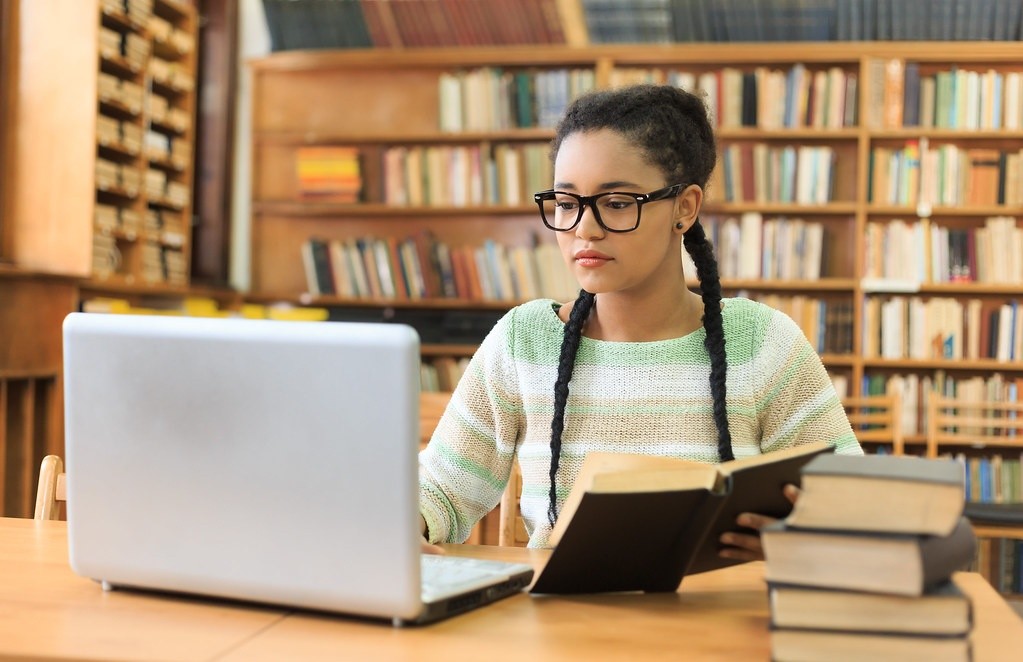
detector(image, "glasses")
[535,183,686,232]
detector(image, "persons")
[419,86,865,560]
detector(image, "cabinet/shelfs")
[239,42,1023,601]
[0,0,200,303]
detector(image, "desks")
[1,517,1023,662]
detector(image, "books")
[93,0,195,283]
[524,439,837,595]
[759,453,980,662]
[295,63,1023,529]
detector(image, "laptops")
[62,313,538,629]
